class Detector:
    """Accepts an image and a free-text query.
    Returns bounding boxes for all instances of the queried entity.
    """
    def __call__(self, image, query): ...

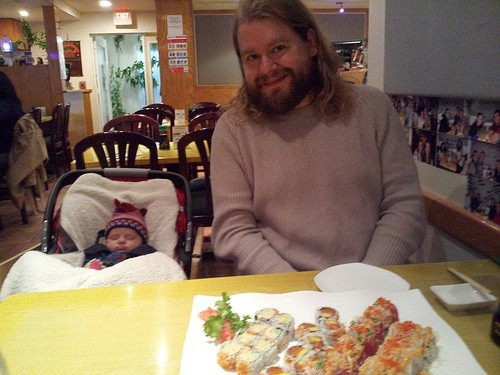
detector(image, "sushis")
[218,297,438,375]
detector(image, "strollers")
[42,166,195,279]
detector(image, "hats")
[104,198,149,243]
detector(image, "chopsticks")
[447,265,492,294]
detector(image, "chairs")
[0,103,223,271]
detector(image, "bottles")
[0,55,5,67]
[159,126,170,151]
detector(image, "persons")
[390,93,500,223]
[78,198,157,271]
[0,71,26,182]
[209,0,428,275]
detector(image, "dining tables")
[0,257,500,375]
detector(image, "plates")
[431,284,497,312]
[180,288,488,375]
[314,263,410,293]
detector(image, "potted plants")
[10,18,47,64]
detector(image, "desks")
[69,141,211,180]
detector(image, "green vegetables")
[204,292,251,338]
